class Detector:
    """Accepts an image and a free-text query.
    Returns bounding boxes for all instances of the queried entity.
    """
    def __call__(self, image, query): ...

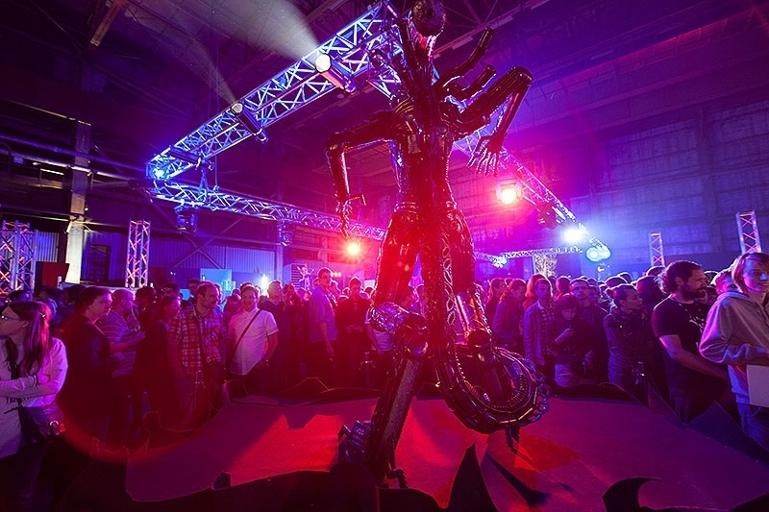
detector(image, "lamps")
[172,206,189,231]
[317,52,357,95]
[277,219,292,249]
[229,98,273,149]
[166,144,217,173]
[189,207,202,233]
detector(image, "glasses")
[0,314,21,322]
[570,285,589,291]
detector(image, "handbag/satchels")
[745,362,769,410]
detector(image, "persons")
[1,252,769,512]
